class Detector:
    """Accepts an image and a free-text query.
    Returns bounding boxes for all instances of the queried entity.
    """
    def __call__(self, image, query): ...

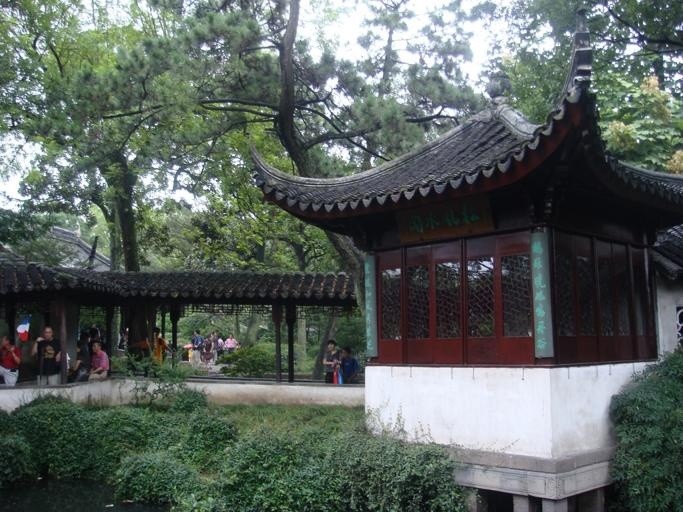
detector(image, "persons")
[322,339,340,382]
[336,345,358,385]
[0,325,245,386]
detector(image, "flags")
[17,316,31,343]
